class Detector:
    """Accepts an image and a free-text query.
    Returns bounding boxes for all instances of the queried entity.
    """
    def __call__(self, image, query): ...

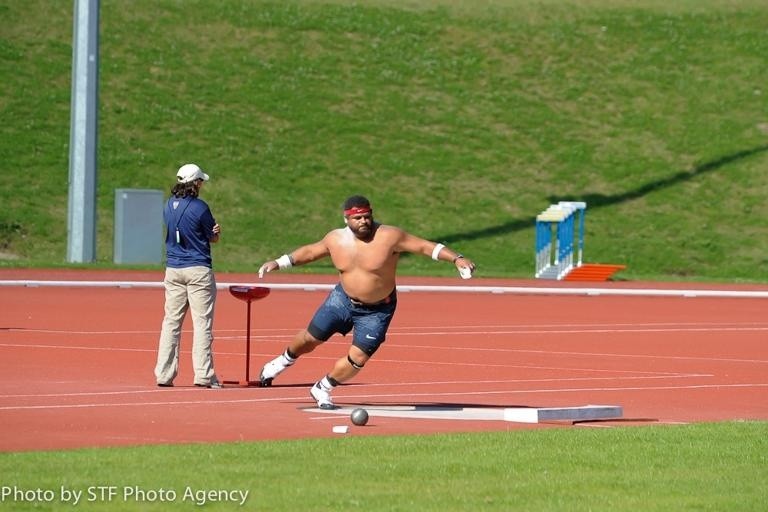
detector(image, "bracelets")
[431,242,446,263]
[275,250,293,272]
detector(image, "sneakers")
[309,381,334,409]
[194,380,223,389]
[260,362,275,387]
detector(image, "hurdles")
[535,202,625,281]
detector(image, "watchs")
[453,253,465,264]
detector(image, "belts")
[351,286,396,307]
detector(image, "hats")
[177,164,209,184]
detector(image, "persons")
[257,193,477,411]
[154,163,227,390]
